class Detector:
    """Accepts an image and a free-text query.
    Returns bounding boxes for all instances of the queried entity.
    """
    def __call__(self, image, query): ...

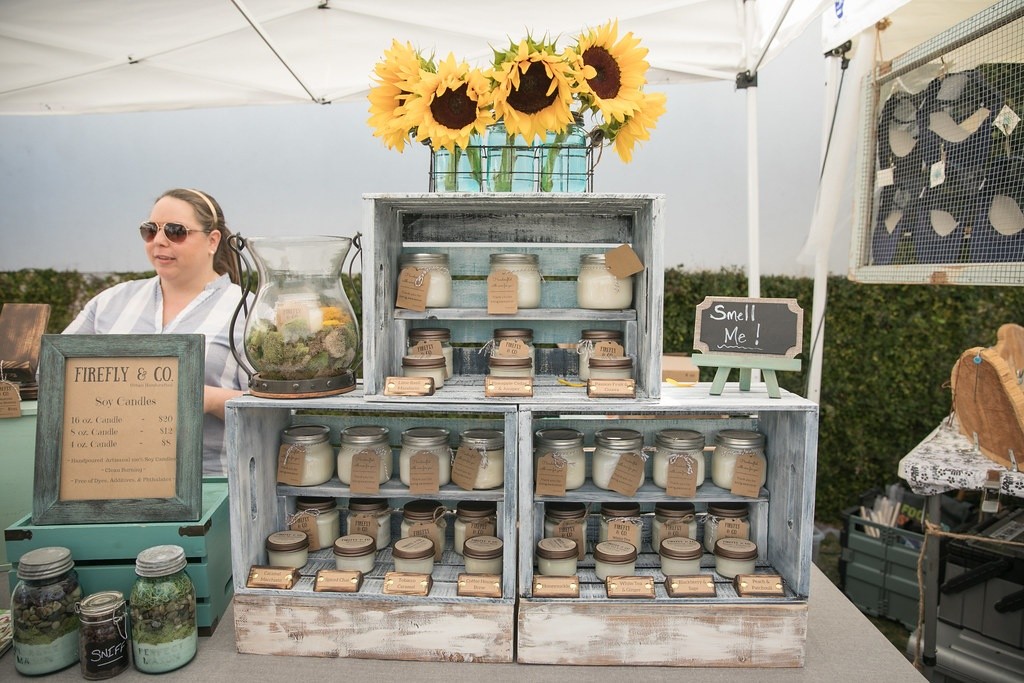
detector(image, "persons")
[28,188,280,477]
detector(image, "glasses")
[138,221,211,246]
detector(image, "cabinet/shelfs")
[224,379,520,664]
[361,192,669,405]
[519,383,819,669]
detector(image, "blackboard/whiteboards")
[693,295,803,358]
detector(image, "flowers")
[367,17,669,187]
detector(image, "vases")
[428,112,590,191]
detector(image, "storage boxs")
[6,475,234,631]
[846,510,927,630]
